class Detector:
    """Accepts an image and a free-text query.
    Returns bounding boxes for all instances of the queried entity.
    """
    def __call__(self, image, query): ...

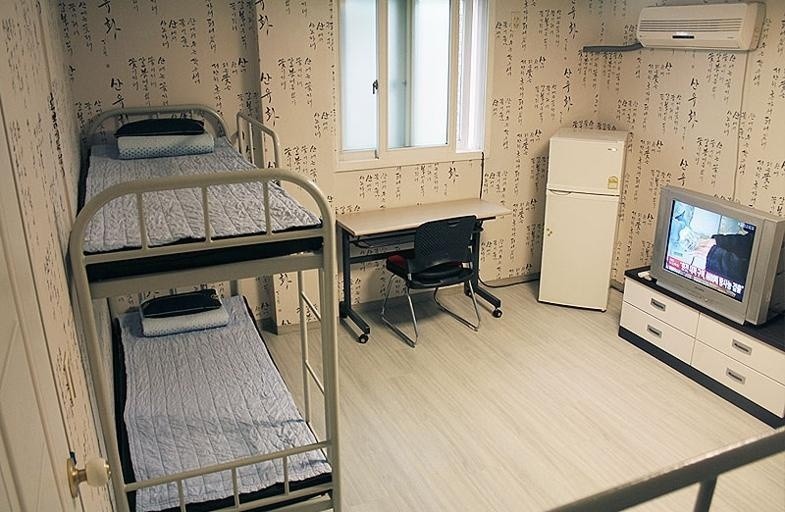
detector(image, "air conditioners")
[636,1,766,56]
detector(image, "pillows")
[141,286,224,317]
[113,116,205,136]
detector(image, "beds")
[65,105,344,512]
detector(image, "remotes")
[644,277,652,281]
[638,270,652,278]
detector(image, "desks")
[334,197,513,343]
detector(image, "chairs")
[382,214,486,349]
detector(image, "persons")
[694,226,754,292]
[669,201,700,257]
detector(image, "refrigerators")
[534,128,630,314]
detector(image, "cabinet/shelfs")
[616,263,785,430]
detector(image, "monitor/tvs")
[650,185,785,325]
